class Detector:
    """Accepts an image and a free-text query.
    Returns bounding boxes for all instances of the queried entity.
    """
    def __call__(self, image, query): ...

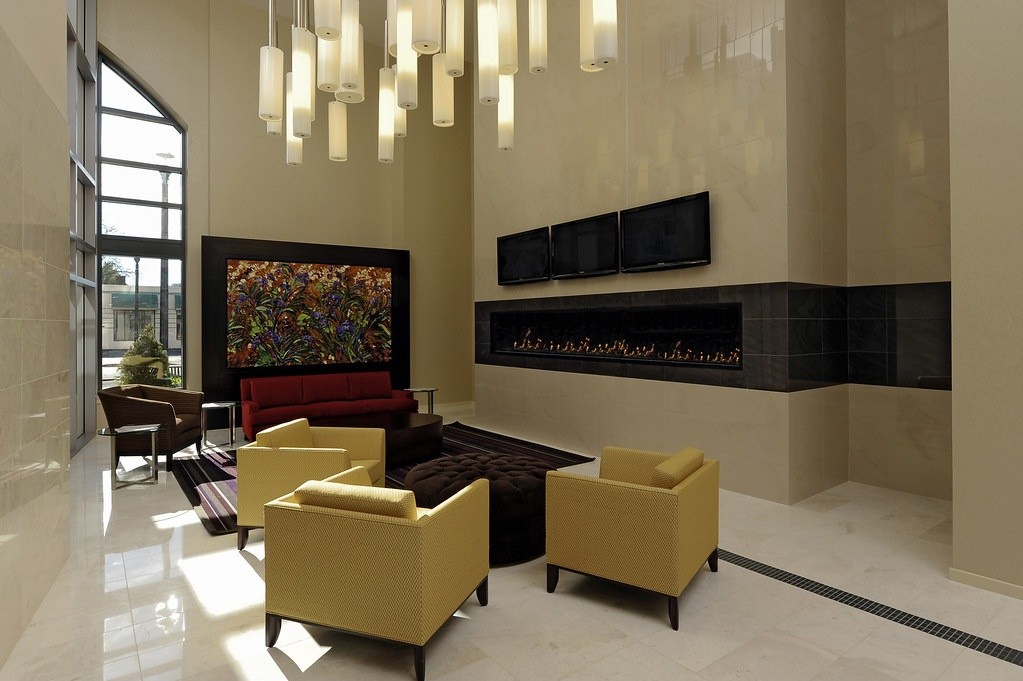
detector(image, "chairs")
[235,418,386,550]
[96,383,204,472]
[545,447,721,630]
[263,465,490,681]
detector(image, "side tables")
[404,386,439,414]
[201,402,237,446]
[99,427,159,491]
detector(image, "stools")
[403,452,558,570]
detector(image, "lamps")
[257,0,621,164]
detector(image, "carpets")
[169,420,596,535]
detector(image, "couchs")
[240,371,419,443]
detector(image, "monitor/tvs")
[496,226,550,285]
[620,191,711,272]
[550,211,620,279]
